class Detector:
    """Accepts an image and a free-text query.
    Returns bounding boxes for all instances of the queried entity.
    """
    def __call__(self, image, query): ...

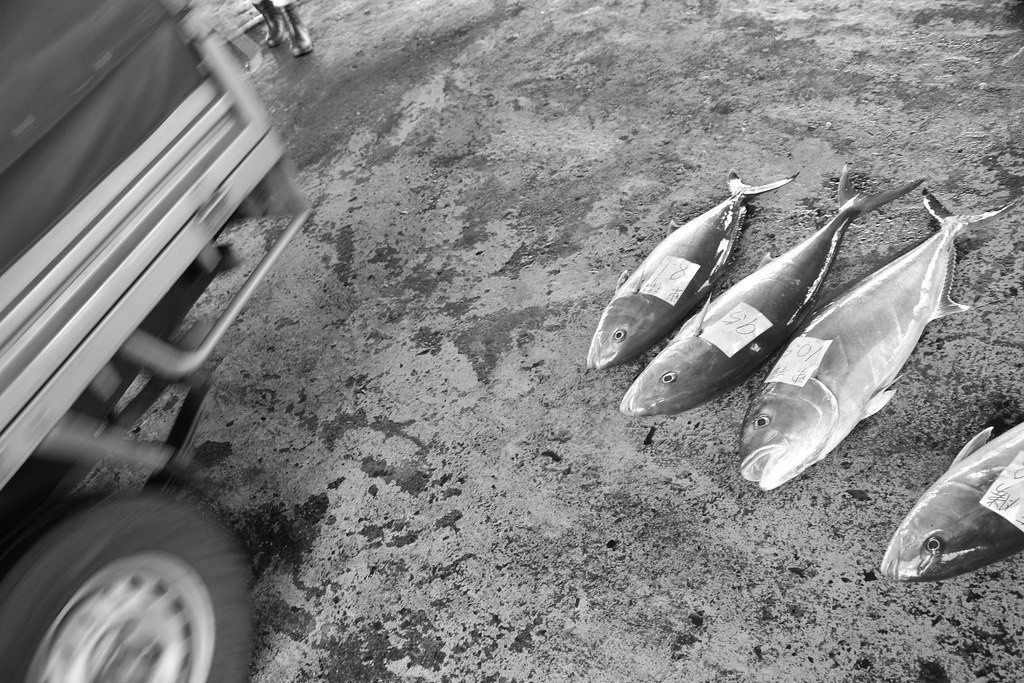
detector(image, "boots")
[252,0,289,47]
[274,1,313,56]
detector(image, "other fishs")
[583,161,1023,584]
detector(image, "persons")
[249,0,313,57]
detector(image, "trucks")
[0,0,313,683]
